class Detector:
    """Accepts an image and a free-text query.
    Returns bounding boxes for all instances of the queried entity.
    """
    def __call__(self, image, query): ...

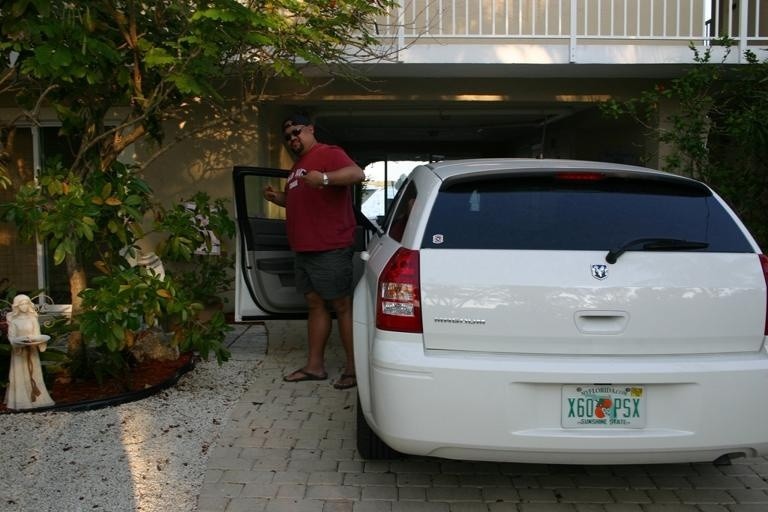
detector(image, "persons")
[261,115,366,389]
[5,294,55,410]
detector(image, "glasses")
[285,126,302,141]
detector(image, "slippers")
[284,368,328,382]
[333,373,356,389]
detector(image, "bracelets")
[321,172,329,186]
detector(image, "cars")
[232,152,767,467]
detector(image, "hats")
[282,115,311,130]
